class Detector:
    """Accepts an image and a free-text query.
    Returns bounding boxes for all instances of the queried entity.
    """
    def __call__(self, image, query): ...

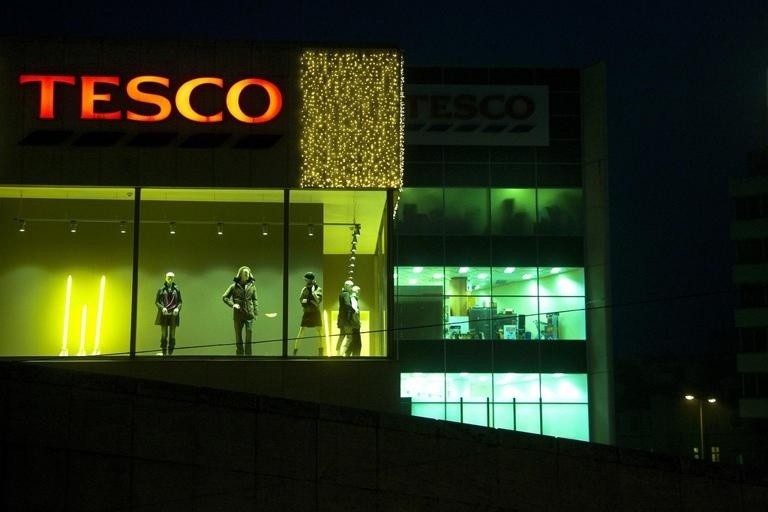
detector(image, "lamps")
[18,222,128,234]
[167,222,317,236]
[350,225,361,274]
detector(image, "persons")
[153,272,182,356]
[334,278,363,357]
[288,270,326,355]
[221,266,259,356]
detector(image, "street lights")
[682,392,719,464]
